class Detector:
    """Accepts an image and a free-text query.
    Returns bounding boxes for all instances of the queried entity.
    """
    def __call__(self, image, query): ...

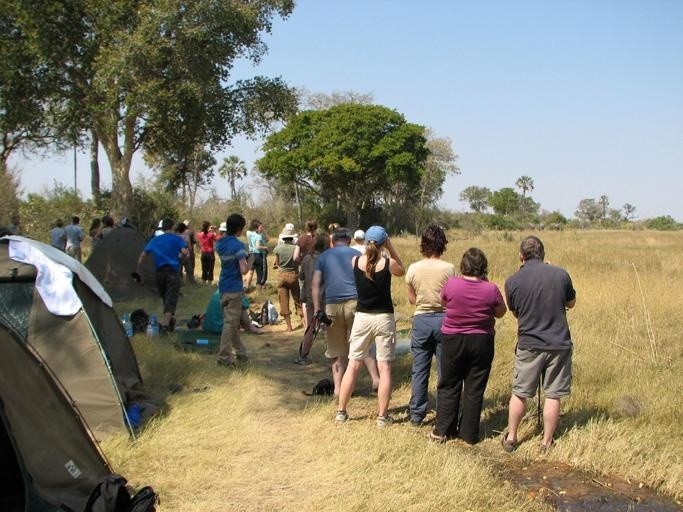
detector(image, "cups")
[196,339,208,345]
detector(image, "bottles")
[262,309,267,325]
[121,314,132,336]
[147,316,159,336]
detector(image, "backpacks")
[83,474,156,512]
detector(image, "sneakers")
[501,432,517,452]
[335,411,348,422]
[378,416,394,427]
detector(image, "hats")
[354,230,364,240]
[293,357,312,364]
[365,226,388,245]
[281,228,298,238]
[219,223,227,230]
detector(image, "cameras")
[316,309,332,326]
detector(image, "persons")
[311,229,378,399]
[213,214,249,368]
[405,225,457,426]
[429,247,506,443]
[51,219,66,251]
[59,217,84,262]
[502,235,576,452]
[89,216,339,335]
[335,227,406,424]
[6,215,20,236]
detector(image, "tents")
[1,315,117,512]
[0,237,145,444]
[83,228,163,299]
